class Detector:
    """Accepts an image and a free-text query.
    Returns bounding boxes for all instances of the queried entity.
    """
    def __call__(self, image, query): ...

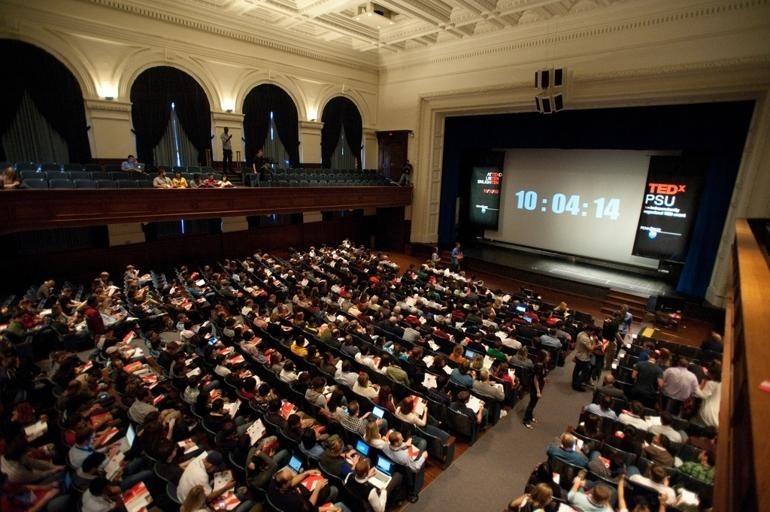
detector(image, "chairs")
[242,161,393,189]
[0,161,235,188]
[510,336,722,510]
[0,244,599,512]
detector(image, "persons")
[203,173,219,188]
[217,174,235,187]
[219,126,237,175]
[188,173,205,188]
[172,169,189,188]
[548,332,725,510]
[251,148,277,177]
[31,158,48,175]
[396,159,413,186]
[0,164,23,190]
[120,154,148,176]
[382,155,392,179]
[572,304,632,391]
[1,240,571,510]
[152,168,174,189]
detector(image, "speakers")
[534,67,564,115]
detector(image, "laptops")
[376,456,393,475]
[114,424,136,454]
[350,440,370,462]
[463,349,475,361]
[368,406,385,422]
[283,455,303,477]
[523,317,532,324]
[516,306,526,312]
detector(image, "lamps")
[105,96,113,100]
[311,118,316,122]
[226,109,232,113]
